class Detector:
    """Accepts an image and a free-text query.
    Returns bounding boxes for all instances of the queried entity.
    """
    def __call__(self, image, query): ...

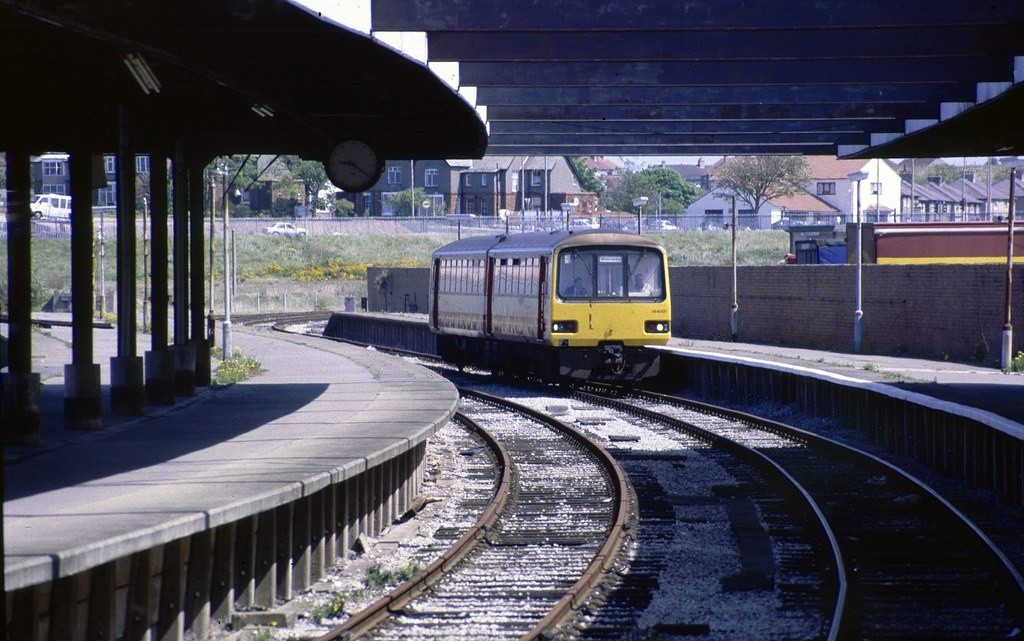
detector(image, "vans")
[31,193,72,220]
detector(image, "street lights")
[504,210,512,235]
[847,171,872,355]
[560,201,575,231]
[631,196,649,236]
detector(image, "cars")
[650,220,681,232]
[570,220,601,229]
[262,223,308,237]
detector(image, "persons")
[630,274,653,297]
[562,277,590,297]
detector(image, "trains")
[429,231,674,382]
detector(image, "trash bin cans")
[344,297,356,312]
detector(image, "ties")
[576,288,578,295]
